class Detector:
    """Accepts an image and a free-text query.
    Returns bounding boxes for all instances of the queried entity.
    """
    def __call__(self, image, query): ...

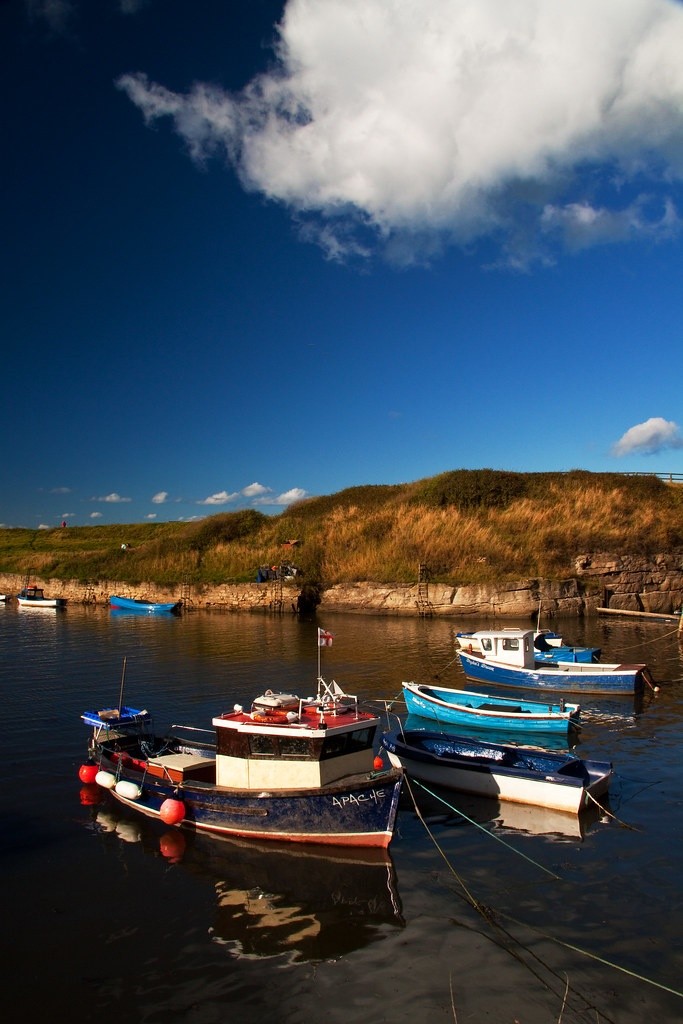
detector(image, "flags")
[318,628,333,647]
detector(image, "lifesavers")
[250,709,299,724]
[306,702,348,714]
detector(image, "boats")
[401,681,581,734]
[362,699,613,814]
[81,800,407,970]
[110,596,183,612]
[455,627,648,694]
[0,593,12,601]
[399,772,589,845]
[78,656,408,850]
[16,585,68,607]
